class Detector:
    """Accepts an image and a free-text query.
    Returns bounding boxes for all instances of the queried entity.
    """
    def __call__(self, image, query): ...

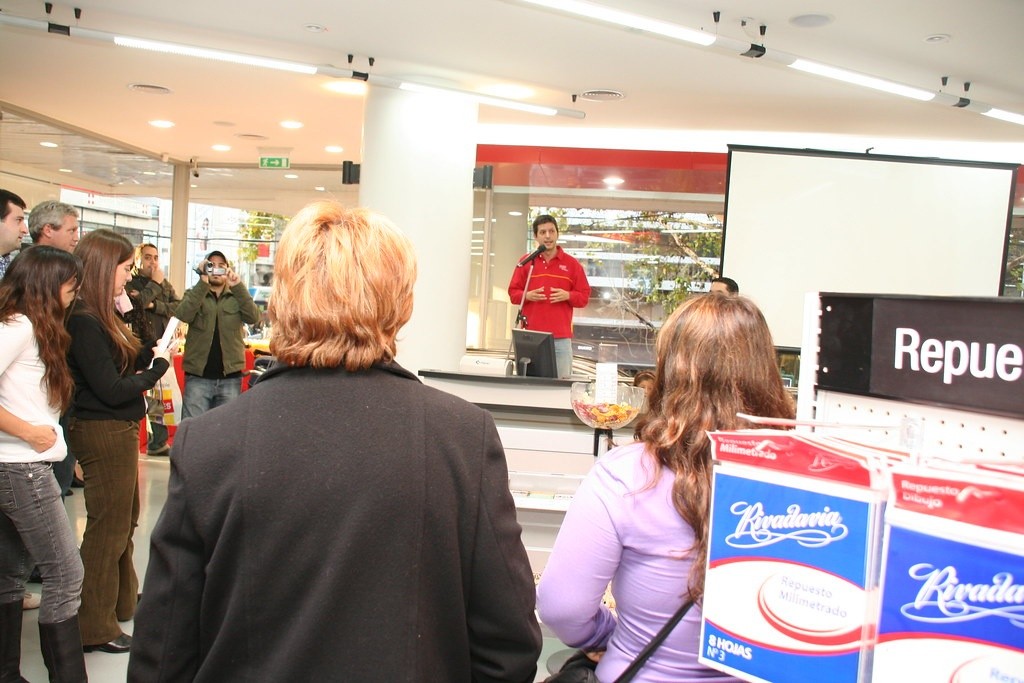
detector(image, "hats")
[204,250,227,263]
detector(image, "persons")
[536,294,798,683]
[175,251,262,420]
[633,369,657,397]
[710,277,739,296]
[127,202,542,683]
[0,189,180,683]
[508,215,592,378]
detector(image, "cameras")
[202,260,226,277]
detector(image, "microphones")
[517,243,546,267]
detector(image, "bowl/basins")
[570,379,645,430]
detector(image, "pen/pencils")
[168,338,180,350]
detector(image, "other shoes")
[70,476,84,487]
[64,490,73,496]
[22,591,41,608]
[148,442,171,456]
[27,570,42,583]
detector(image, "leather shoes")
[83,633,134,653]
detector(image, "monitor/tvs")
[511,326,557,378]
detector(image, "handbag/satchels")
[144,380,164,418]
[543,641,606,683]
